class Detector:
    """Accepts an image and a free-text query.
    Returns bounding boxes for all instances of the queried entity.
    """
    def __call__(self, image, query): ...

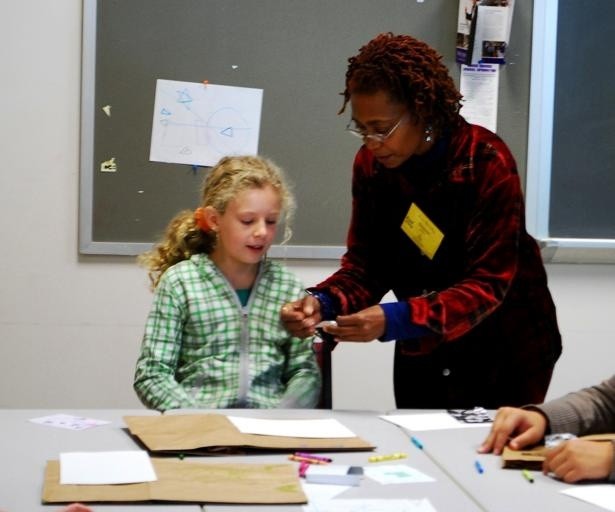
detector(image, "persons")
[281,32,564,408]
[477,375,615,482]
[133,156,321,410]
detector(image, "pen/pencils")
[367,435,423,463]
[475,459,483,473]
[288,452,332,477]
[522,468,534,483]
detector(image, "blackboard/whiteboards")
[77,0,615,266]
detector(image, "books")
[500,433,615,471]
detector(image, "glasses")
[345,112,410,143]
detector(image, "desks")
[1,408,615,512]
